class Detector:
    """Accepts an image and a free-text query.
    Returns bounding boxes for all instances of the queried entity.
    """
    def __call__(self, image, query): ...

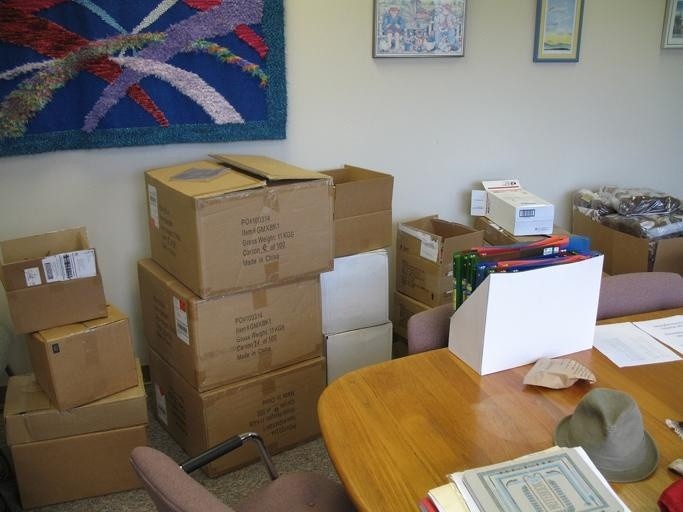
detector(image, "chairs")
[597,271,681,319]
[407,304,455,353]
[128,433,354,512]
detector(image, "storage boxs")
[571,186,683,275]
[470,179,569,248]
[1,225,154,508]
[320,164,393,388]
[396,214,483,354]
[137,153,334,479]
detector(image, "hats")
[552,386,660,483]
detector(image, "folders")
[453,236,602,316]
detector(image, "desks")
[317,306,682,512]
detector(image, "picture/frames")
[659,0,682,52]
[533,0,583,63]
